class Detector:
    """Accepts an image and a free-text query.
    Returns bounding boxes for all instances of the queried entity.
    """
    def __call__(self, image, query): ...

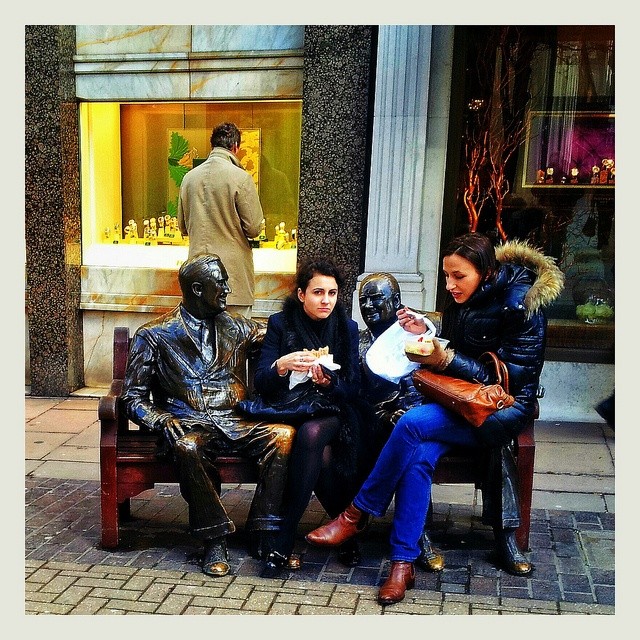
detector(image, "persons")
[255,260,362,575]
[177,122,264,323]
[304,233,564,605]
[120,253,301,576]
[358,270,546,577]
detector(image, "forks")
[404,310,428,321]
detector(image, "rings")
[312,377,319,382]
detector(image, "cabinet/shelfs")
[522,110,615,190]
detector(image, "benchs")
[98,326,538,553]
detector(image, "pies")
[303,346,329,358]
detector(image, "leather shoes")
[378,562,415,604]
[333,541,361,567]
[265,535,295,570]
[303,501,369,547]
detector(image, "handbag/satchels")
[411,351,515,428]
[236,364,343,424]
